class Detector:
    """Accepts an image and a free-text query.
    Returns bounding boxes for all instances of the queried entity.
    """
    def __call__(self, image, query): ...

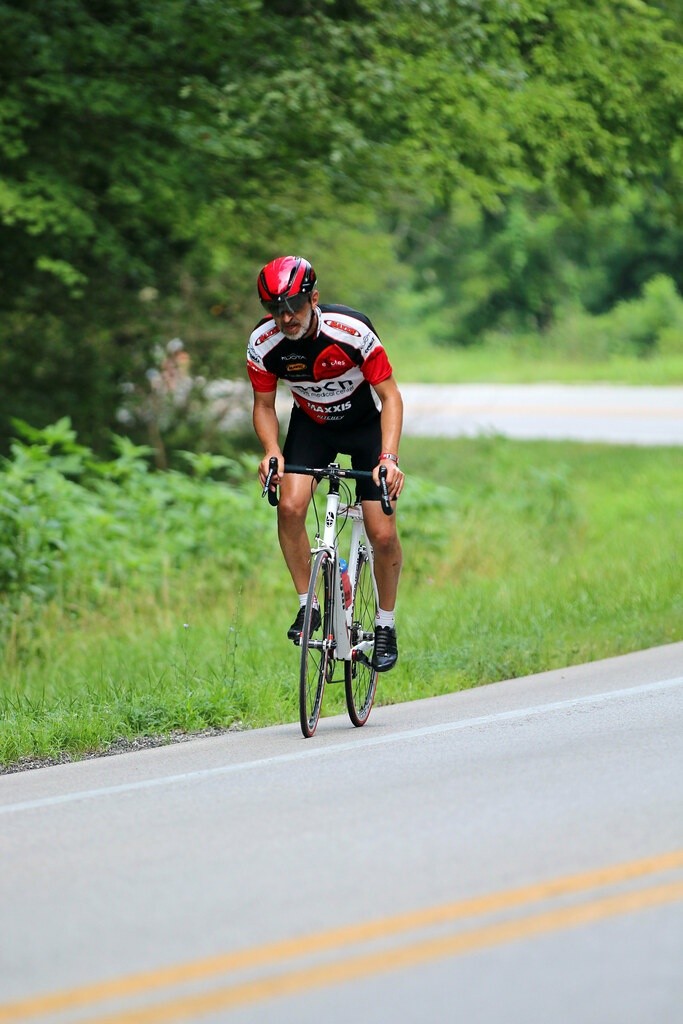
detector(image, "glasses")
[261,293,309,316]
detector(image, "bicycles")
[262,457,398,738]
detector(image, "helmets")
[258,255,316,302]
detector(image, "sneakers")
[372,625,398,671]
[287,605,321,640]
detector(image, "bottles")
[339,558,352,608]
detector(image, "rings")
[396,481,399,484]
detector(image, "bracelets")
[378,453,399,466]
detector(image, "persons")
[247,256,405,672]
